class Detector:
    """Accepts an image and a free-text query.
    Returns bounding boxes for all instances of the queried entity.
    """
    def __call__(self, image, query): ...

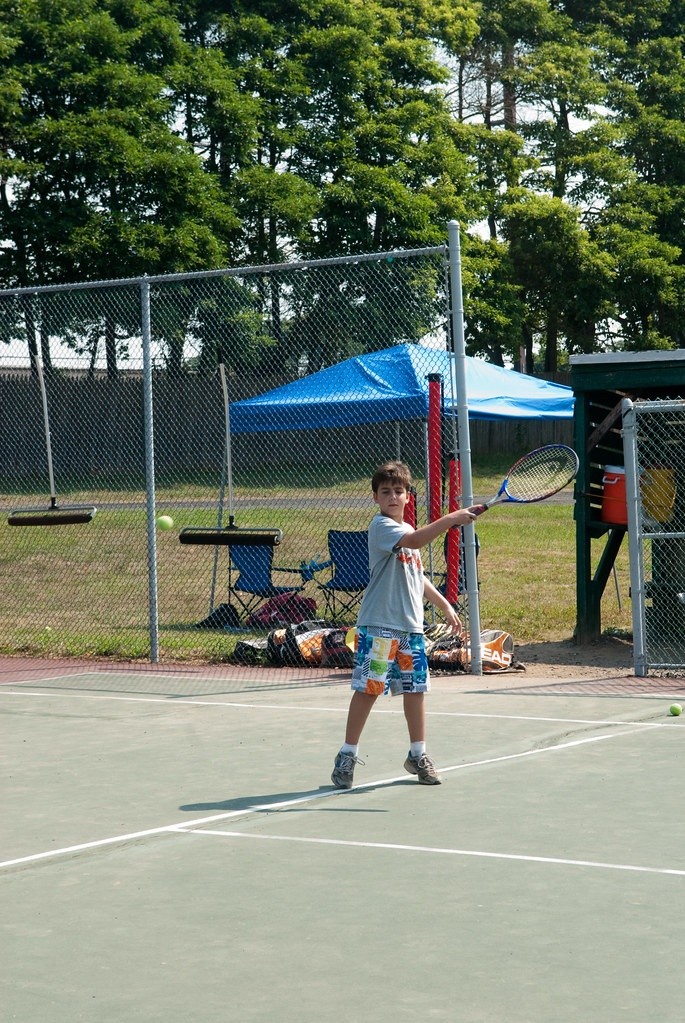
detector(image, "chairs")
[418,527,482,635]
[316,529,371,621]
[230,542,305,625]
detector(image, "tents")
[225,341,574,629]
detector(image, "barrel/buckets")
[602,464,628,524]
[640,470,675,522]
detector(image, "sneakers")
[403,750,443,785]
[330,747,357,788]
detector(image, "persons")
[331,461,483,790]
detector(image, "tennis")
[670,703,681,715]
[156,515,173,531]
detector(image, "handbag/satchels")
[236,619,515,675]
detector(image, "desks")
[230,557,337,617]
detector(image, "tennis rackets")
[449,444,579,528]
